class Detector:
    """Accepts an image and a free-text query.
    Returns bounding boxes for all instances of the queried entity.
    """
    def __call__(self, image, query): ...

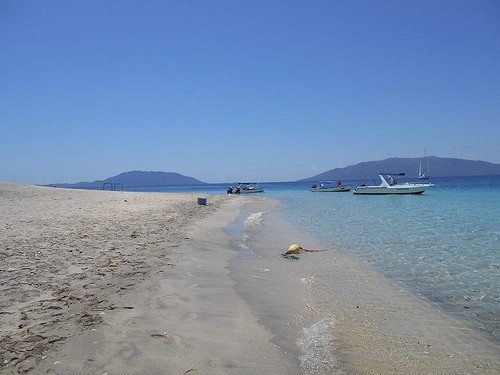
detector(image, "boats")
[227,183,264,193]
[308,185,352,192]
[353,172,436,195]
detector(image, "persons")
[336,181,341,187]
[313,184,316,188]
[389,177,393,185]
